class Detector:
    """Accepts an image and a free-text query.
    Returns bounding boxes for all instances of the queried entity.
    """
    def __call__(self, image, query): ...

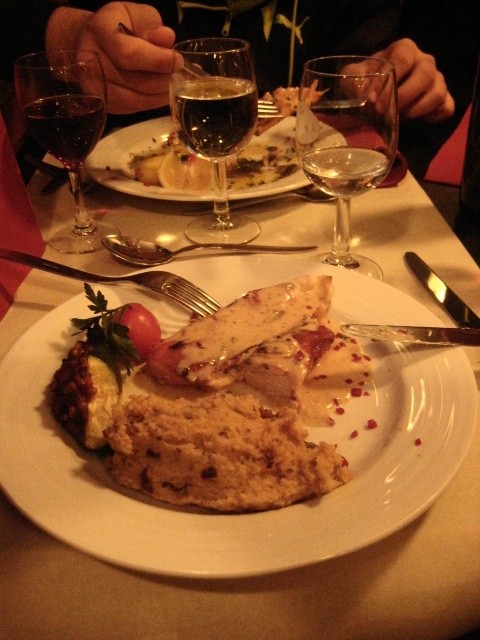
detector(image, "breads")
[107,391,350,513]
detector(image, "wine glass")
[13,48,121,255]
[166,36,261,246]
[294,53,400,284]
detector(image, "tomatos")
[111,305,163,363]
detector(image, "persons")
[1,0,480,184]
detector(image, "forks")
[0,248,222,318]
[117,22,283,120]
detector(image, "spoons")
[101,234,319,267]
[181,184,340,217]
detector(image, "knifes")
[404,251,479,329]
[309,96,369,110]
[340,322,479,348]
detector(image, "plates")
[0,253,480,581]
[83,111,349,204]
